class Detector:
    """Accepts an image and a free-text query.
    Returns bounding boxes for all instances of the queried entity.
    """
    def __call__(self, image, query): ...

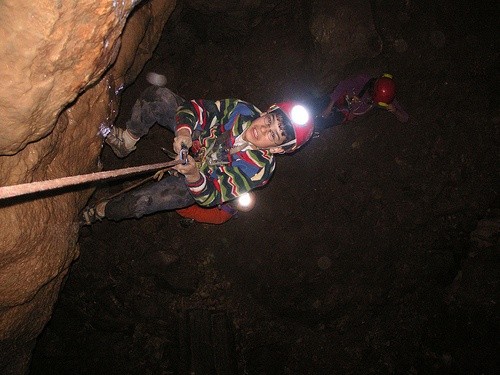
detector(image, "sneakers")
[106,125,136,157]
[80,201,100,225]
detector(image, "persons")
[77,85,314,228]
[314,72,409,135]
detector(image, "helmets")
[269,100,314,153]
[372,77,396,107]
